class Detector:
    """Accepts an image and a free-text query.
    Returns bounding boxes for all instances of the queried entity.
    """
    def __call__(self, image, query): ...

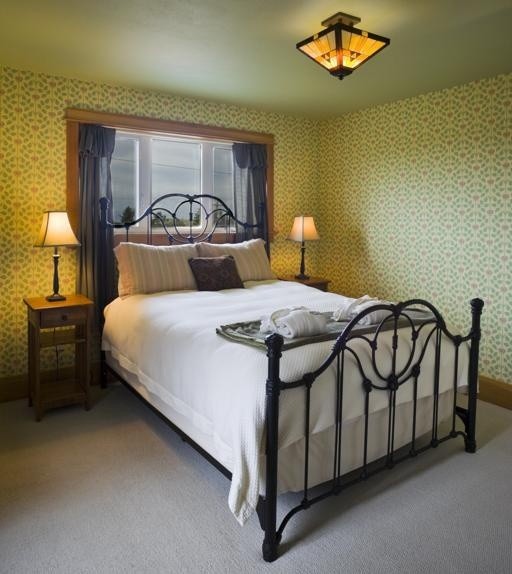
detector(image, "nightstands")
[278,274,331,292]
[22,295,94,420]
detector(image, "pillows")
[188,254,244,291]
[113,242,198,300]
[196,238,276,281]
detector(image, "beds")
[98,194,484,562]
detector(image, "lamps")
[285,215,320,280]
[33,211,82,300]
[296,11,390,79]
